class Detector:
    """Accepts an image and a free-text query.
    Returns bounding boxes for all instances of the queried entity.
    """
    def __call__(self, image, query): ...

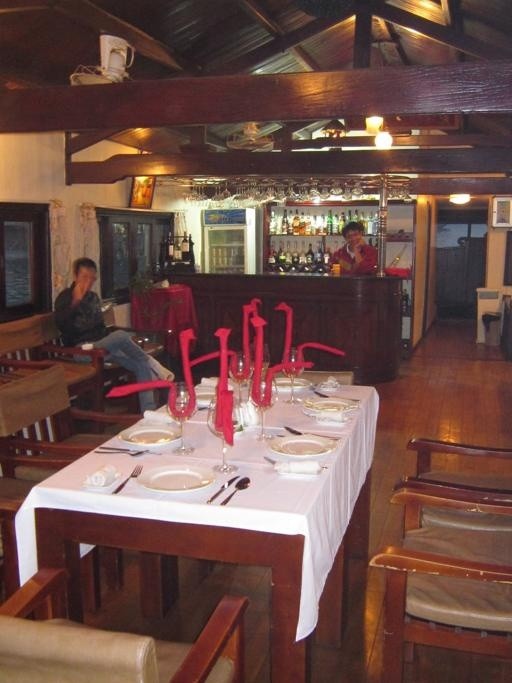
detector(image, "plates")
[136,464,217,493]
[119,424,183,446]
[268,432,337,458]
[261,374,314,392]
[305,394,358,414]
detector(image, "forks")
[112,464,144,497]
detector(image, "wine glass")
[185,179,414,207]
[167,340,304,474]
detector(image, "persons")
[54,257,176,414]
[327,221,377,274]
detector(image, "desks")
[130,284,195,357]
[13,376,378,682]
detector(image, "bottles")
[390,244,408,268]
[401,288,409,312]
[166,230,189,262]
[267,207,378,273]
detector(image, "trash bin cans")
[482,311,501,345]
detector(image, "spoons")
[93,450,149,457]
[219,476,251,506]
[314,390,361,403]
[284,425,342,440]
[263,456,327,471]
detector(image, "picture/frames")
[130,175,158,211]
[489,197,510,228]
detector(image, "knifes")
[207,474,241,503]
[98,445,160,456]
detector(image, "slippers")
[159,366,176,382]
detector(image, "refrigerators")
[200,207,256,276]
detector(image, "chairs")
[1,448,102,622]
[0,364,141,597]
[393,426,512,528]
[1,319,105,432]
[49,311,140,412]
[102,299,172,367]
[369,490,512,683]
[2,565,249,682]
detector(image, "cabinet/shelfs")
[263,200,416,321]
